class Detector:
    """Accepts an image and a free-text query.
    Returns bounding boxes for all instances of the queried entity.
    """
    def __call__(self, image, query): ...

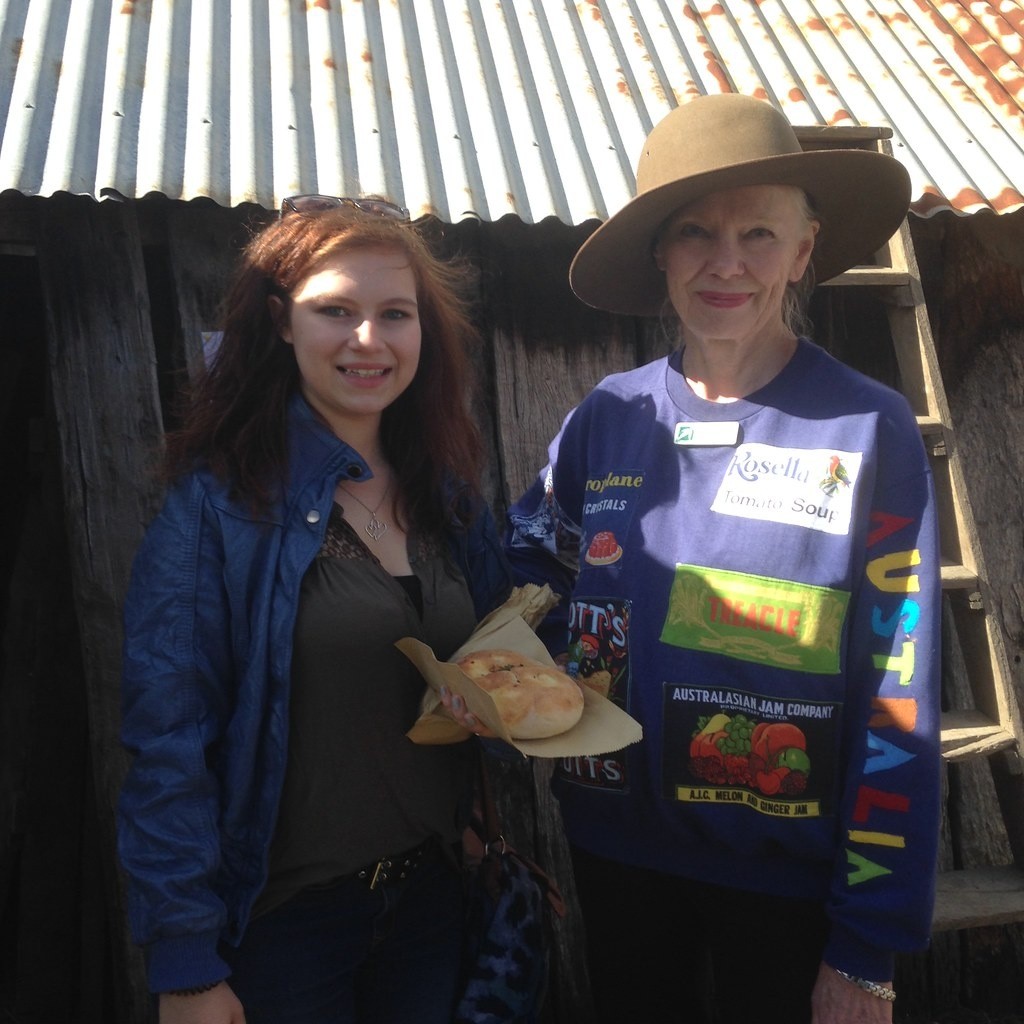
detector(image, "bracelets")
[831,967,897,1003]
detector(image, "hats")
[570,93,912,317]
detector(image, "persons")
[111,198,598,1024]
[504,95,943,1024]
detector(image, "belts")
[298,837,434,902]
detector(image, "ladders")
[791,125,1023,933]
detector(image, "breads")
[459,650,585,740]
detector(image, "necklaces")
[334,473,394,541]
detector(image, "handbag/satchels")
[455,841,567,1024]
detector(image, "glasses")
[279,191,411,228]
